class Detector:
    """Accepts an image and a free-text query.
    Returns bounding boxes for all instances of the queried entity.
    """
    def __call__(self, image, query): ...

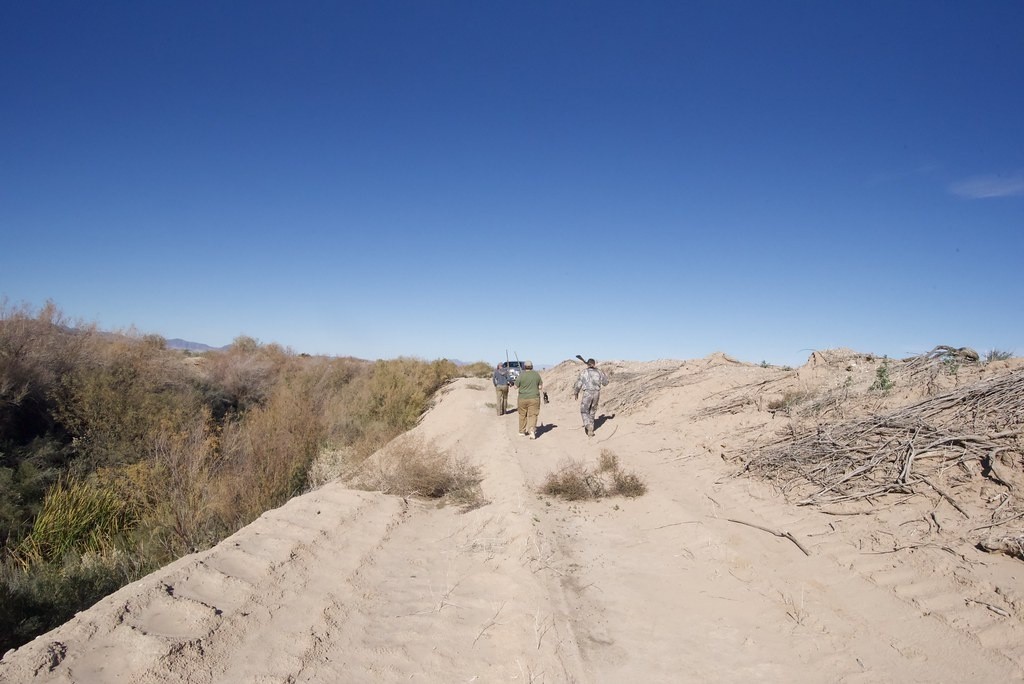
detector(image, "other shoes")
[519,431,535,439]
[586,424,594,438]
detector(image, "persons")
[514,360,543,440]
[492,362,514,417]
[573,357,609,437]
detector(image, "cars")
[504,361,525,384]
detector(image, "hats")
[524,360,532,366]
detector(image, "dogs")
[543,392,549,404]
